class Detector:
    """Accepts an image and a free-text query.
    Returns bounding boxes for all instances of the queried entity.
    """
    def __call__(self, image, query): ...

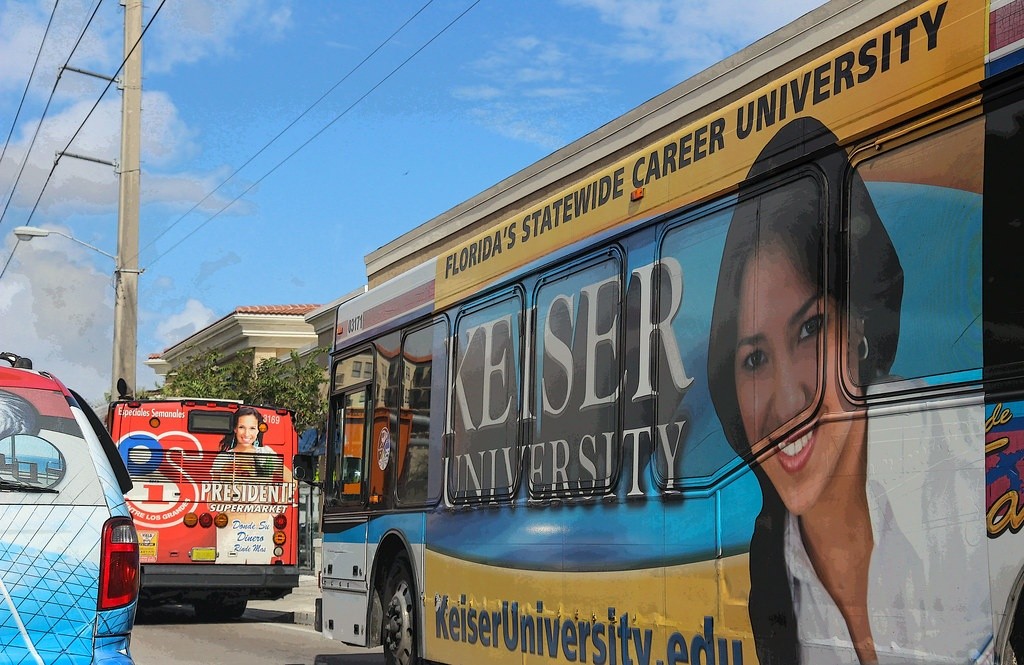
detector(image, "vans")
[0,366,140,665]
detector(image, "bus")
[91,378,301,620]
[296,0,1024,664]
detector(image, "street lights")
[14,226,139,400]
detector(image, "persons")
[208,407,297,564]
[707,118,1024,665]
